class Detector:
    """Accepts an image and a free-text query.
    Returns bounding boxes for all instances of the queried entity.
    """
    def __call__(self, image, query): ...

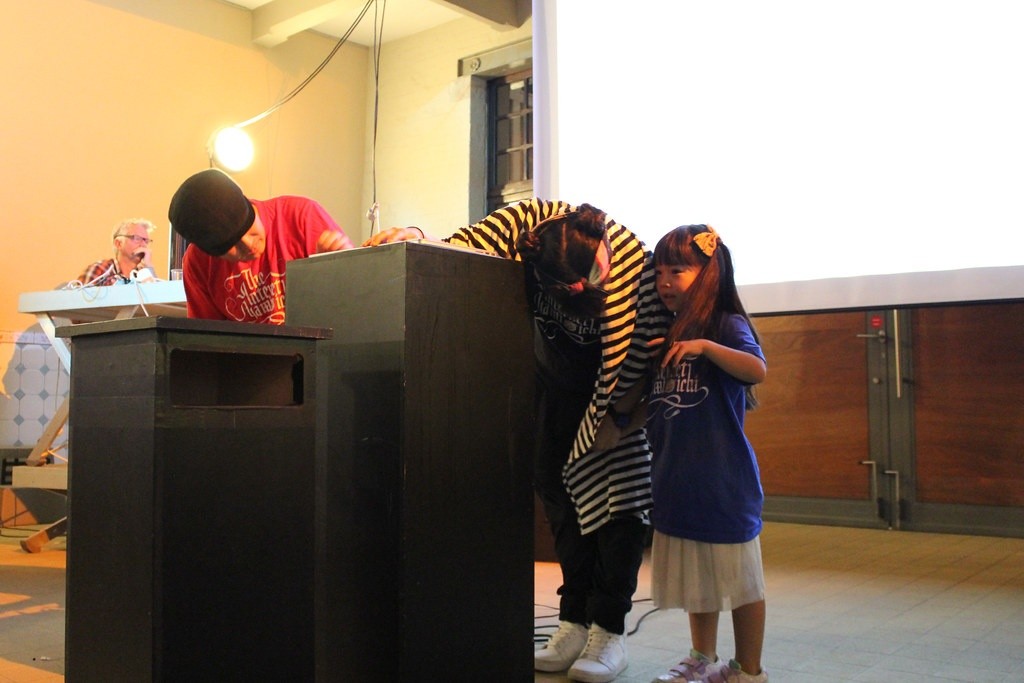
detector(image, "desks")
[12,279,188,554]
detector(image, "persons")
[363,198,767,683]
[168,170,356,326]
[77,217,157,286]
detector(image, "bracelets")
[407,226,425,239]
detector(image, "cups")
[171,269,182,280]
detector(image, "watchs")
[607,404,631,428]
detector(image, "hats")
[168,169,255,256]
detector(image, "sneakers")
[651,652,723,683]
[535,620,591,672]
[691,658,767,683]
[567,621,627,682]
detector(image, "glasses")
[114,234,154,246]
